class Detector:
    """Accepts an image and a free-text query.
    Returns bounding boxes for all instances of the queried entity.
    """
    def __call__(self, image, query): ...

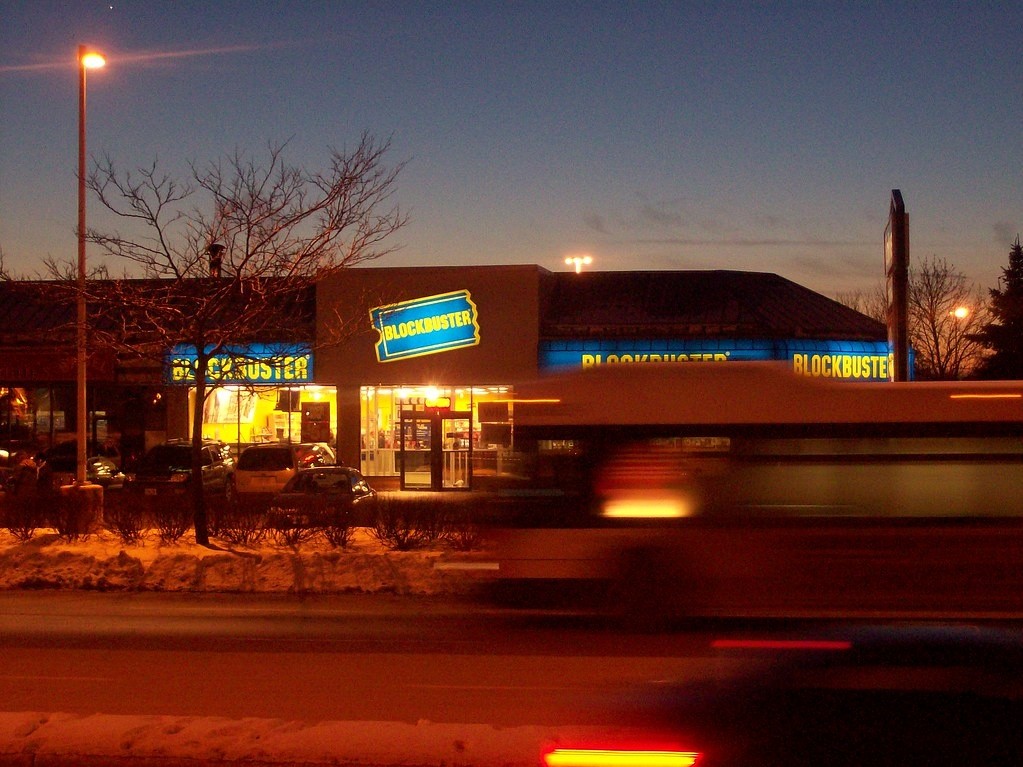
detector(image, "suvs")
[122,437,235,511]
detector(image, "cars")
[267,466,377,514]
[32,439,121,468]
[46,455,127,479]
[225,441,337,505]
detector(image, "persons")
[6,452,46,485]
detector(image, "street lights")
[73,44,106,485]
[949,307,967,372]
